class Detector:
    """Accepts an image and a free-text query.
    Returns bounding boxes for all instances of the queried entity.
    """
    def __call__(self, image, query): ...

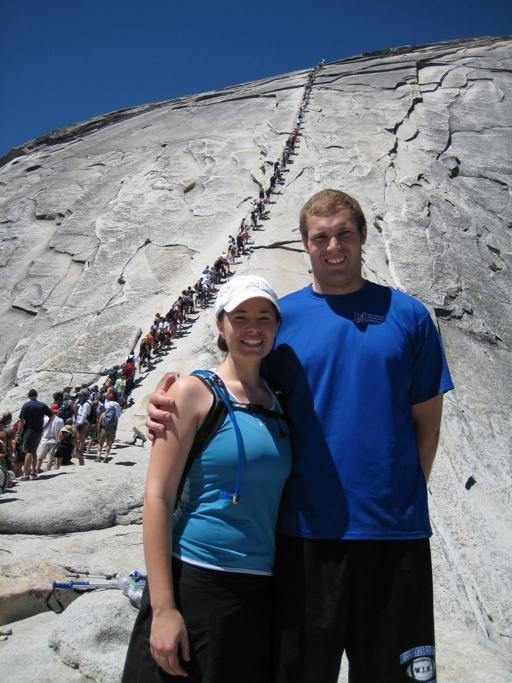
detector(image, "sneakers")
[12,472,39,481]
[77,446,108,463]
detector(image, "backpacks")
[102,406,118,430]
[85,400,97,425]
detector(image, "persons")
[146,189,454,683]
[0,61,312,496]
[120,275,294,681]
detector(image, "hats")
[61,400,71,408]
[214,274,281,322]
[50,402,58,413]
[76,389,91,399]
[63,384,73,389]
